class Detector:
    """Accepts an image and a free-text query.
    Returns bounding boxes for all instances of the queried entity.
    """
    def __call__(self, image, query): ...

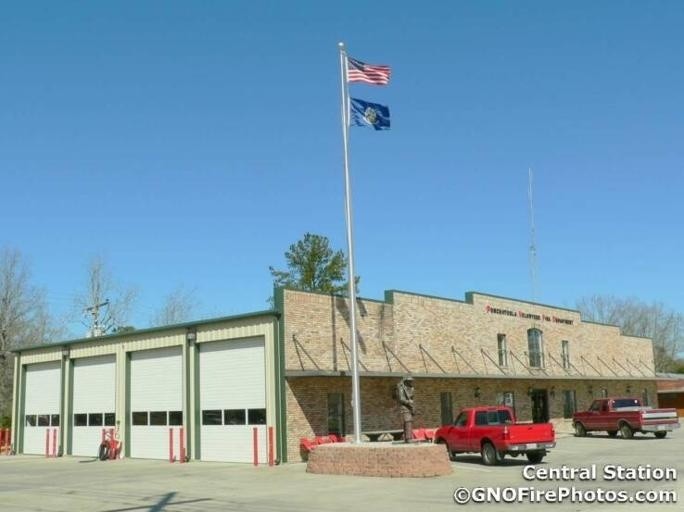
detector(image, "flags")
[346,54,391,86]
[347,96,391,131]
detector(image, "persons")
[397,376,415,443]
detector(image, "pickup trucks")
[433,407,556,467]
[574,397,679,441]
